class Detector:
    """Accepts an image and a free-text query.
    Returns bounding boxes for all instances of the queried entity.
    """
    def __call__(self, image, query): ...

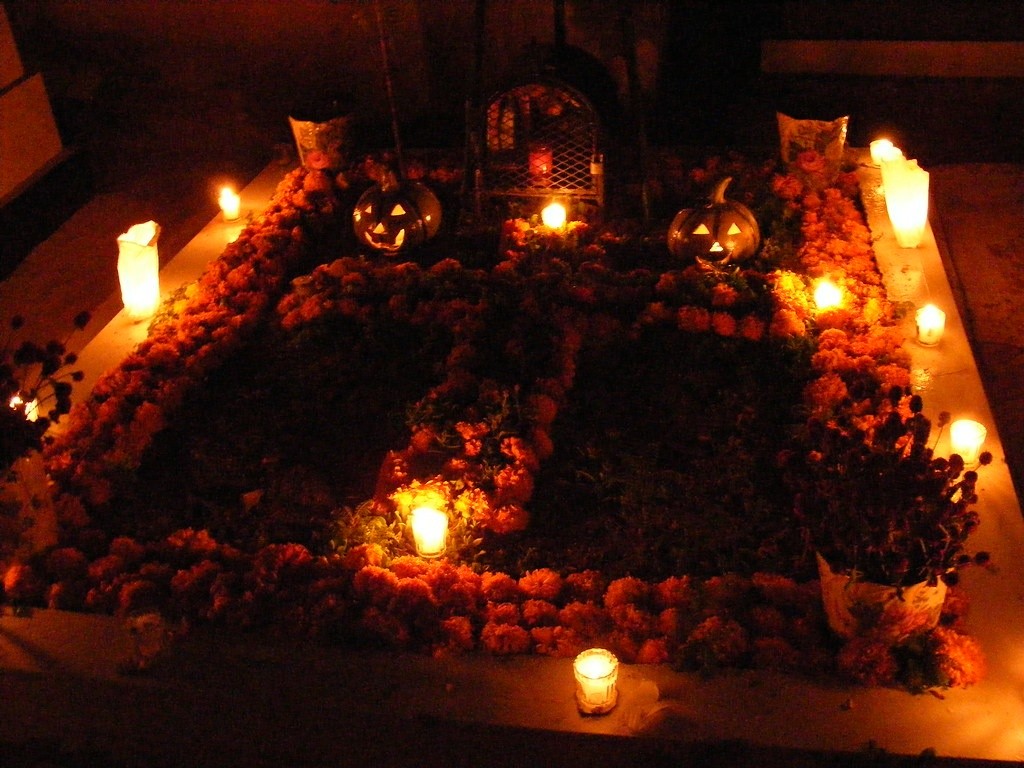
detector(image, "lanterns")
[354,163,442,257]
[666,177,760,267]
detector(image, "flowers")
[0,149,987,696]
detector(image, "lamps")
[881,156,932,248]
[116,221,162,323]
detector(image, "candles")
[917,304,945,346]
[411,505,448,559]
[543,204,569,235]
[573,648,620,717]
[951,418,986,464]
[218,185,244,221]
[870,136,905,167]
